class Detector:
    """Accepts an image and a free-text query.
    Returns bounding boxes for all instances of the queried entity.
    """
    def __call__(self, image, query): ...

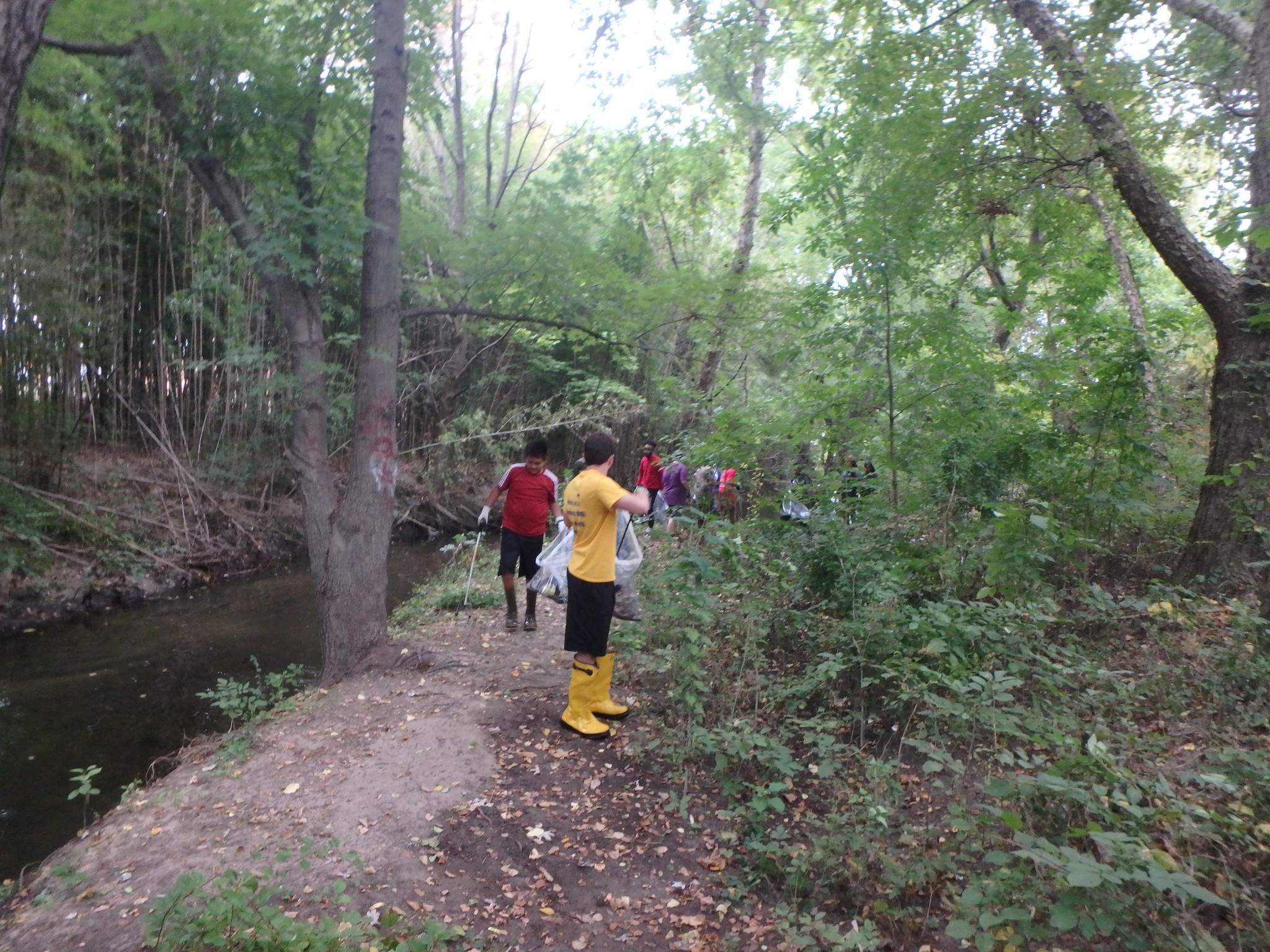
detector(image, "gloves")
[477,506,491,527]
[634,486,649,495]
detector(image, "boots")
[561,651,629,740]
[504,585,537,632]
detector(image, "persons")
[477,440,568,631]
[696,449,879,535]
[635,440,665,533]
[661,450,691,539]
[572,454,587,477]
[556,431,651,741]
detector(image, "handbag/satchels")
[612,509,643,621]
[525,526,574,604]
